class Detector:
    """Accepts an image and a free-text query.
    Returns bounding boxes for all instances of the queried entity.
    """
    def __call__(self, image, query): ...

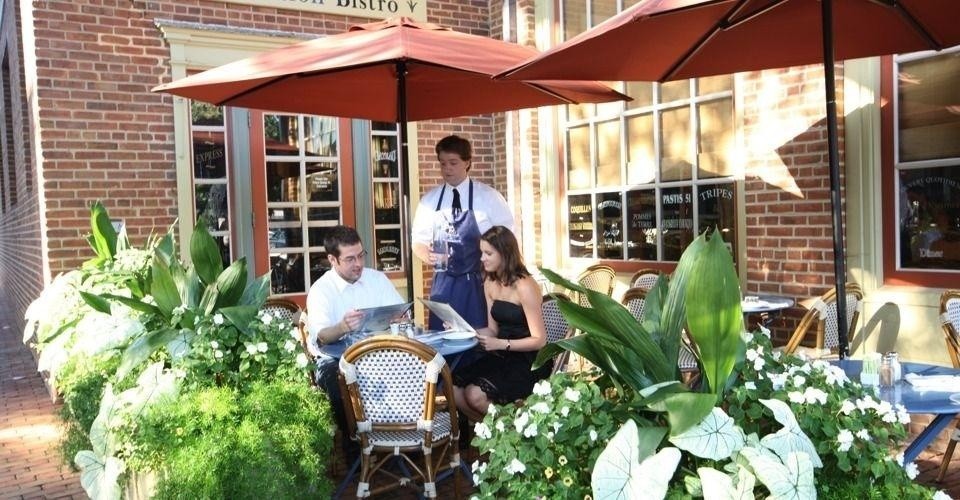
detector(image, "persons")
[446,224,555,424]
[303,223,417,475]
[409,135,518,334]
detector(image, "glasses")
[336,251,367,263]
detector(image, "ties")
[452,189,462,219]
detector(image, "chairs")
[337,333,464,498]
[255,264,868,389]
[932,287,959,486]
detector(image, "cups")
[389,323,399,336]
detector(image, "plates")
[441,330,476,342]
[365,313,392,331]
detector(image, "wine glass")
[602,222,619,246]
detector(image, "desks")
[813,356,959,467]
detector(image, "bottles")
[400,323,416,339]
[430,215,449,274]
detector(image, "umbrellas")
[151,11,636,334]
[488,1,960,363]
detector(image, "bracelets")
[505,336,513,356]
